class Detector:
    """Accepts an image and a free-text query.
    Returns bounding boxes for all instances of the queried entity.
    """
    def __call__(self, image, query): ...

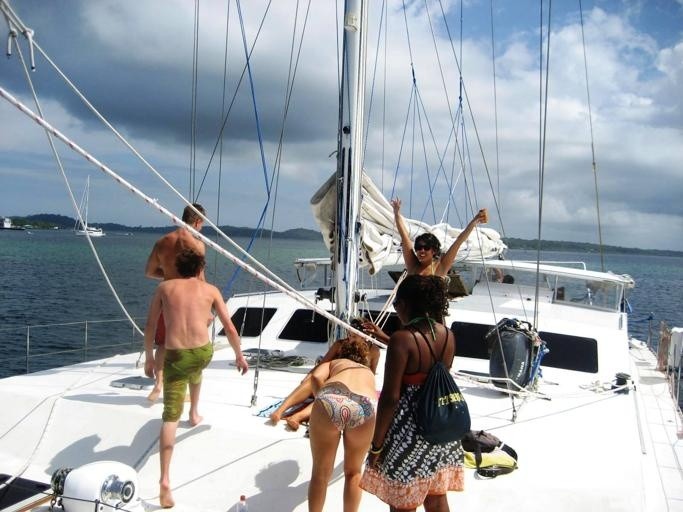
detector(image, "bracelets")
[368,447,384,454]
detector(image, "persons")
[490,267,506,284]
[270,316,379,431]
[502,275,514,284]
[145,249,248,507]
[305,339,377,511]
[388,195,488,278]
[368,274,458,511]
[145,203,215,403]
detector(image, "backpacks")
[412,325,471,442]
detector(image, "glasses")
[415,243,433,251]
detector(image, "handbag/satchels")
[460,430,500,453]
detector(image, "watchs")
[370,440,384,451]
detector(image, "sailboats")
[0,1,682,512]
[72,175,106,238]
